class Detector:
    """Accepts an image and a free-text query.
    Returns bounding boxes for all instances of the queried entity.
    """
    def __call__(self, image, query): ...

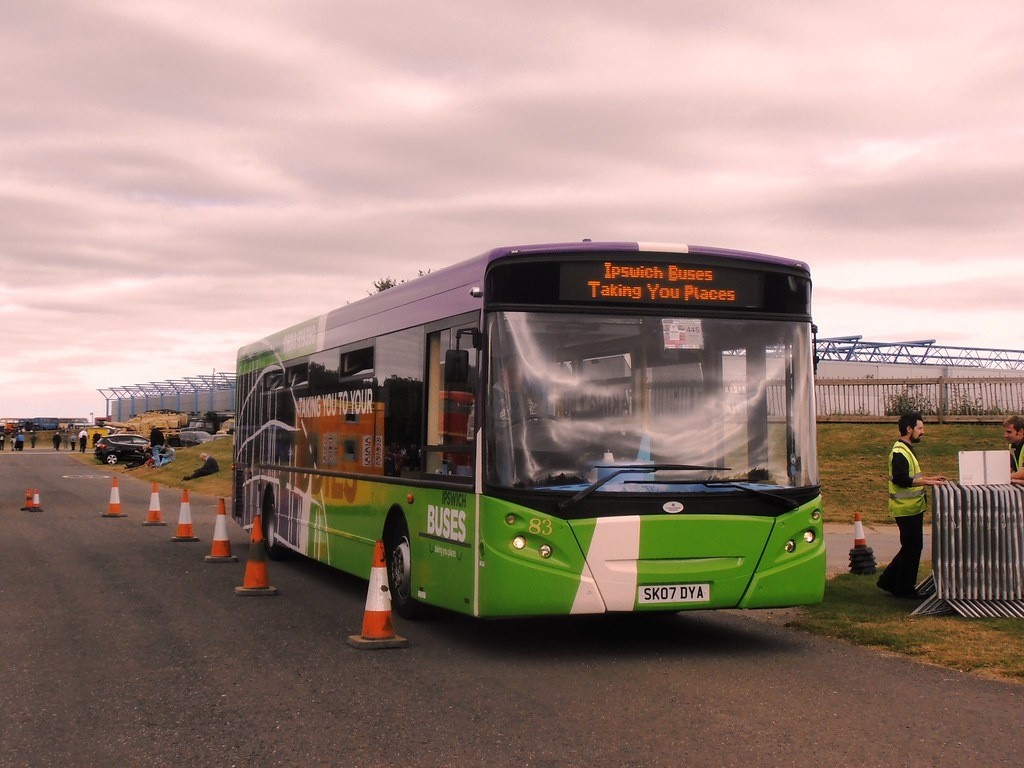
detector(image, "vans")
[180,430,210,448]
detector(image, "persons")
[192,453,221,478]
[93,429,101,447]
[876,412,952,600]
[11,429,70,453]
[78,427,89,453]
[70,431,78,450]
[127,444,154,469]
[158,444,177,468]
[150,424,165,468]
[1,431,6,450]
[1004,413,1024,485]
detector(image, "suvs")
[94,434,153,465]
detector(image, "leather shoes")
[877,574,895,592]
[892,585,930,599]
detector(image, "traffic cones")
[142,483,168,527]
[348,541,410,647]
[21,488,31,513]
[850,511,877,579]
[171,489,199,543]
[101,476,128,518]
[204,498,240,561]
[31,489,43,512]
[234,512,279,596]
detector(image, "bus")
[230,242,827,621]
[0,417,112,434]
